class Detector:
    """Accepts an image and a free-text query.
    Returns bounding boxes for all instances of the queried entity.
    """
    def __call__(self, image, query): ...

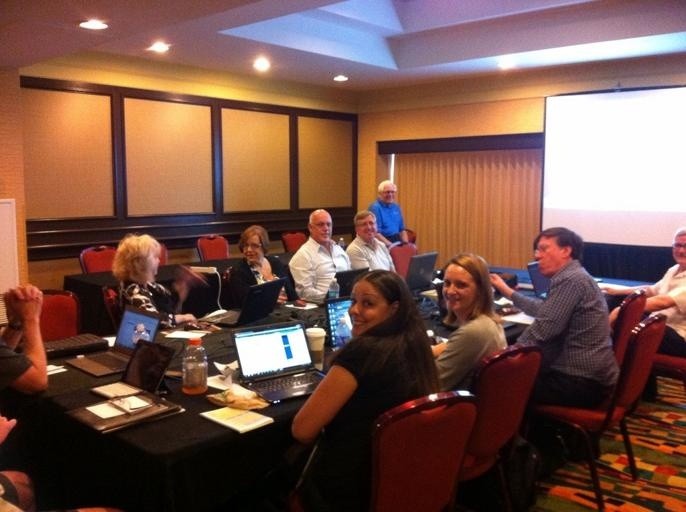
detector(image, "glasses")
[242,243,262,250]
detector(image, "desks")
[62,252,293,336]
[30,290,626,511]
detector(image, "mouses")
[183,321,202,331]
[294,299,307,307]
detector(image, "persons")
[0,415,124,512]
[1,285,47,396]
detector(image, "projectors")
[488,270,518,301]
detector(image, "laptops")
[64,310,161,379]
[406,253,439,290]
[436,283,517,330]
[197,278,285,328]
[88,339,176,402]
[324,297,354,352]
[527,261,551,299]
[305,267,369,307]
[230,320,328,405]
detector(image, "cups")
[304,327,327,372]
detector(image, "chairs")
[525,313,666,511]
[100,285,123,335]
[39,290,79,343]
[197,235,230,261]
[611,289,686,383]
[78,244,119,273]
[282,232,307,252]
[456,342,542,482]
[372,389,480,512]
[390,227,420,280]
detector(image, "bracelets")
[7,322,24,330]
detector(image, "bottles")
[425,330,440,359]
[179,337,209,395]
[328,277,341,300]
[338,237,345,250]
